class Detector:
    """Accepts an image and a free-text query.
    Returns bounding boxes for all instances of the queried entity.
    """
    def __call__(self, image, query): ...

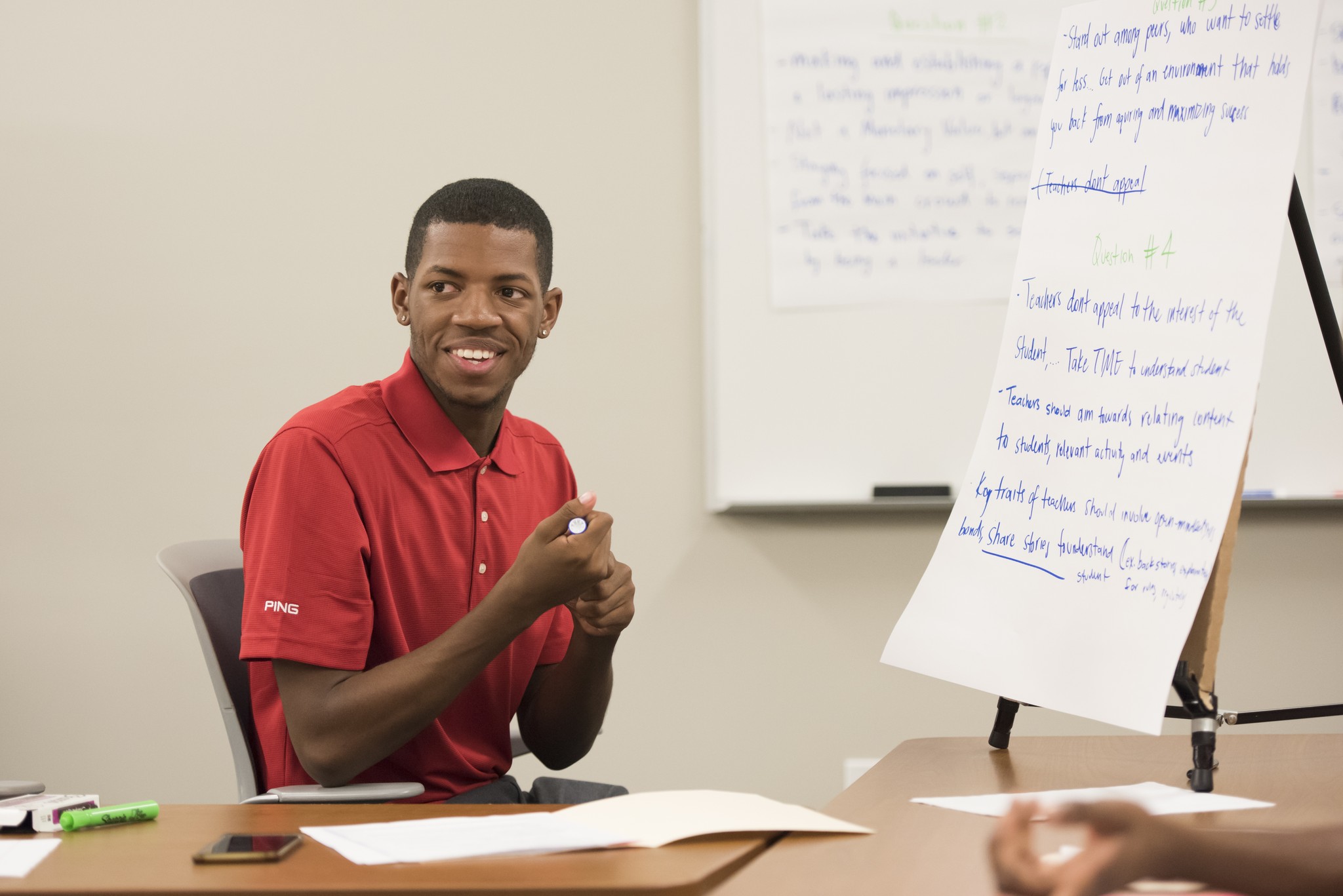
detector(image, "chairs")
[160,540,536,805]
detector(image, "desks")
[714,726,1340,896]
[0,803,780,895]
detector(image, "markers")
[1242,489,1275,501]
[1333,490,1343,499]
[693,1,1343,515]
[59,800,158,832]
[568,517,587,535]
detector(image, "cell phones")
[191,833,300,864]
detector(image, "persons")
[239,176,641,801]
[983,796,1343,896]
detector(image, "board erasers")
[873,486,953,503]
